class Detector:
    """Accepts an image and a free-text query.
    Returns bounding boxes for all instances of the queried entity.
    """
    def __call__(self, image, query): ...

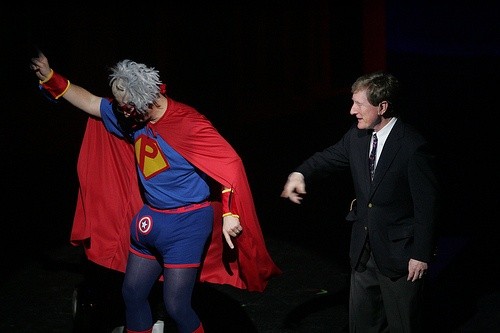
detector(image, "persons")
[31,53,282,333]
[282,73,437,333]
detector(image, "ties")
[368,133,378,183]
